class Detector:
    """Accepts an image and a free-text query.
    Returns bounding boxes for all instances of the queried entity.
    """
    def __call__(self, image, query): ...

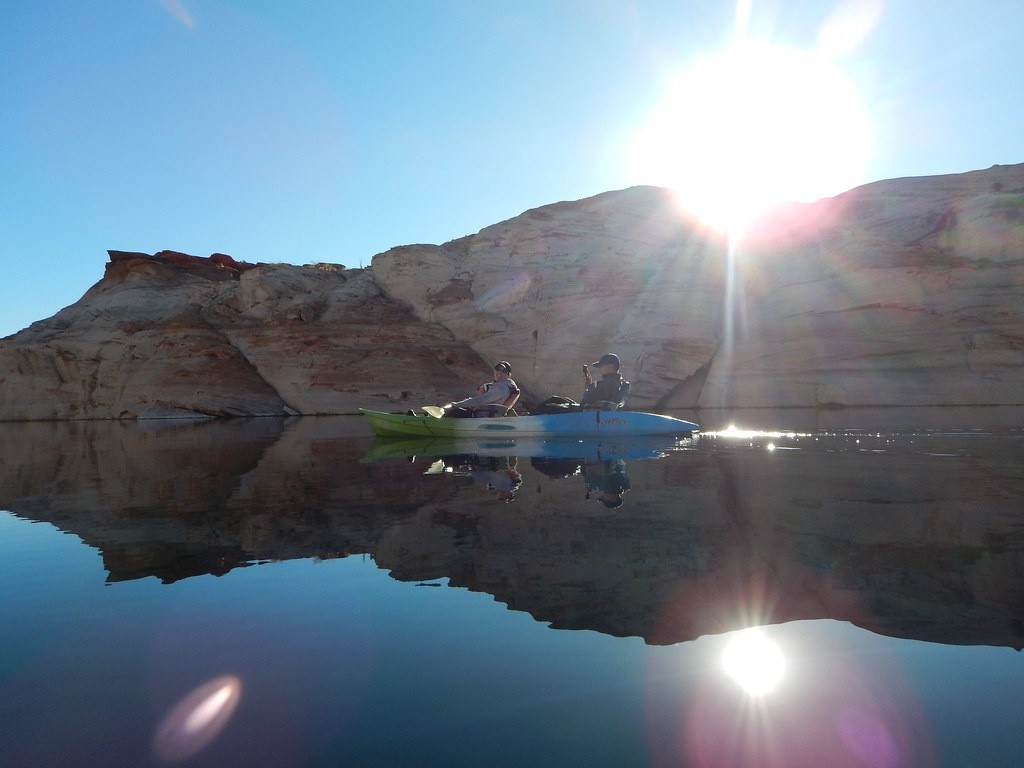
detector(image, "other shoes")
[407,409,415,416]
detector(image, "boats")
[358,433,699,464]
[360,407,701,437]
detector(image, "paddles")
[420,398,472,420]
[583,364,592,380]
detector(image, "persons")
[528,353,629,416]
[581,460,631,509]
[408,456,521,503]
[407,361,520,418]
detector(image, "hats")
[495,361,511,371]
[592,354,619,367]
